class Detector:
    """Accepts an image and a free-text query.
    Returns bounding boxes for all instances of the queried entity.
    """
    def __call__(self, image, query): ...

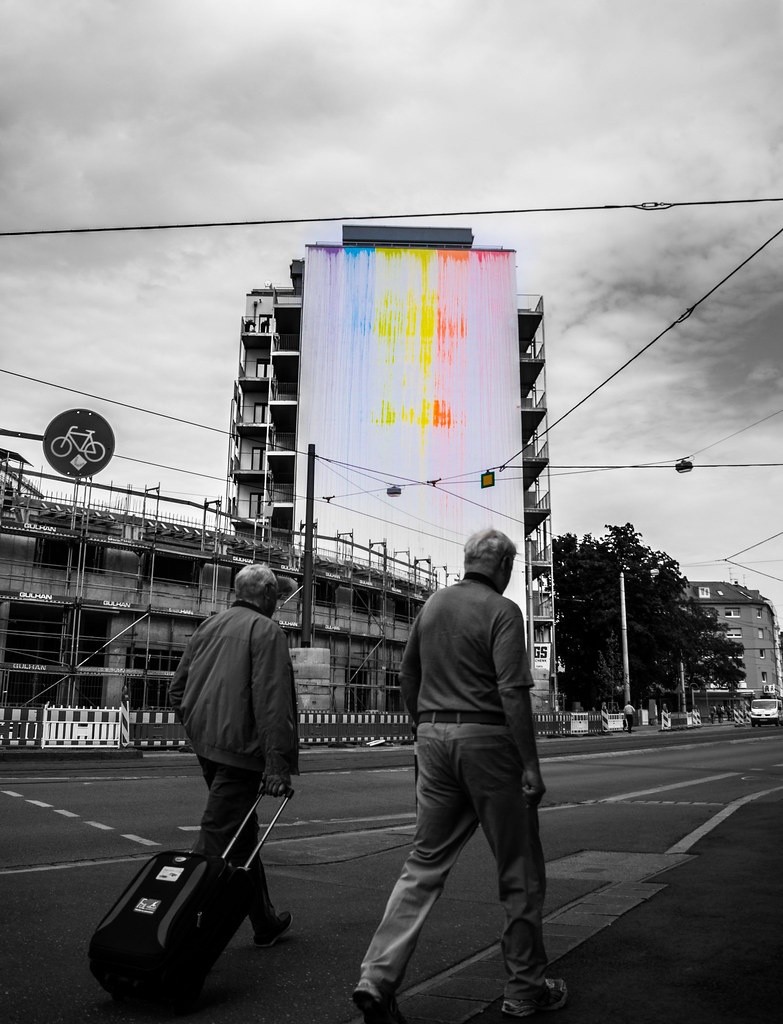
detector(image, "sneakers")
[352,977,407,1024]
[259,910,294,947]
[500,978,568,1017]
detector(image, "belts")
[418,710,506,725]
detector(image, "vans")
[750,699,782,727]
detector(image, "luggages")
[88,780,294,1018]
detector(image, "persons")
[352,529,566,1024]
[622,701,636,734]
[168,564,301,946]
[709,704,730,723]
